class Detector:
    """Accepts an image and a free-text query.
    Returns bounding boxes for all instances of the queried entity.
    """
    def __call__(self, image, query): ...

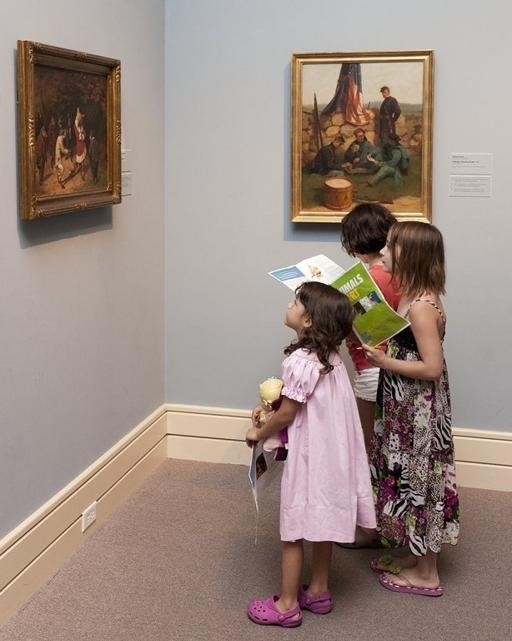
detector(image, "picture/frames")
[289,49,435,226]
[16,40,121,222]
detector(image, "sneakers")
[335,527,403,549]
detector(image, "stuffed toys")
[257,377,288,462]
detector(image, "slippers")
[372,556,442,597]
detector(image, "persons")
[332,204,398,555]
[249,281,380,627]
[361,132,410,188]
[311,134,344,176]
[379,86,402,144]
[341,127,375,171]
[354,221,461,597]
[34,103,103,186]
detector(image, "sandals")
[298,584,333,615]
[248,596,302,628]
